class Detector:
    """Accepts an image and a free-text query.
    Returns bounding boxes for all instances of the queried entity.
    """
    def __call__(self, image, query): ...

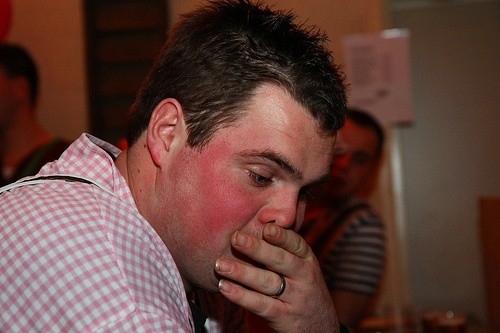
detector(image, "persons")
[0,0,347,333]
[300,107,387,333]
[1,41,71,191]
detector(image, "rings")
[273,273,286,297]
